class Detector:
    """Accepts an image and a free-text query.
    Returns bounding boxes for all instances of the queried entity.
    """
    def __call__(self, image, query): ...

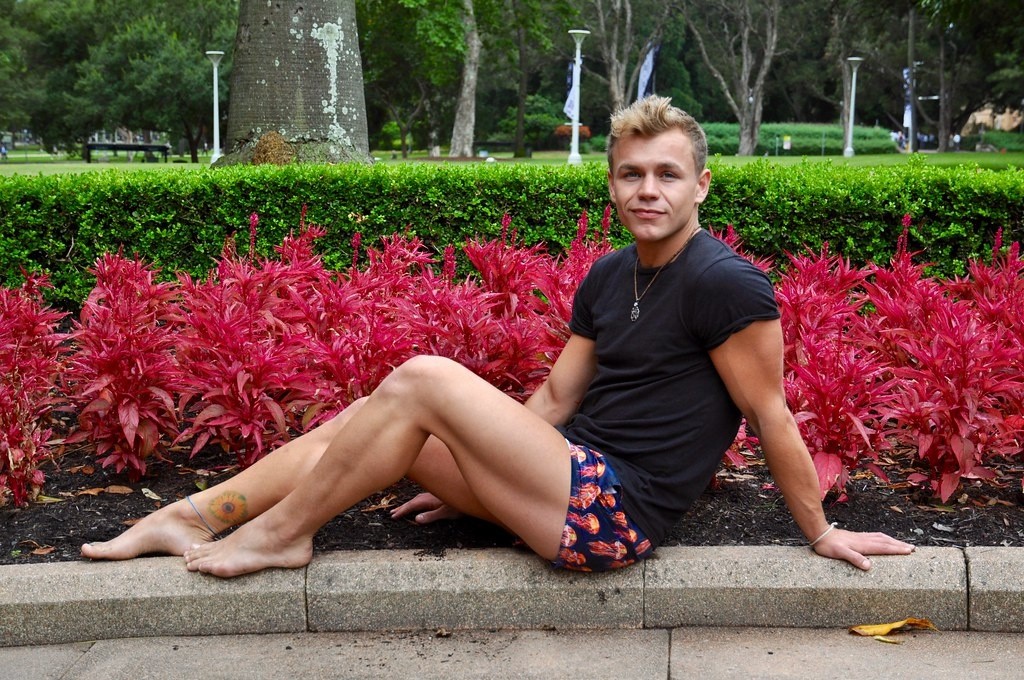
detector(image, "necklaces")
[630,226,700,321]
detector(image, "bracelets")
[810,522,837,545]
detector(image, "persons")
[890,129,962,152]
[82,93,915,578]
